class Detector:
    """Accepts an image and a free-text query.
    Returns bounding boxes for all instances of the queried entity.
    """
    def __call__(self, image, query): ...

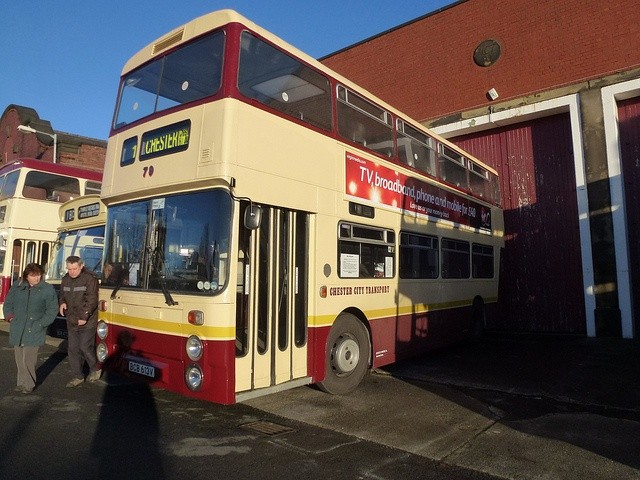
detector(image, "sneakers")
[66,379,84,388]
[86,369,102,383]
[14,385,32,394]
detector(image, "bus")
[44,194,203,341]
[0,157,105,320]
[95,9,505,406]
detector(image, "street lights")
[16,124,58,164]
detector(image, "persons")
[58,256,102,387]
[3,263,59,394]
